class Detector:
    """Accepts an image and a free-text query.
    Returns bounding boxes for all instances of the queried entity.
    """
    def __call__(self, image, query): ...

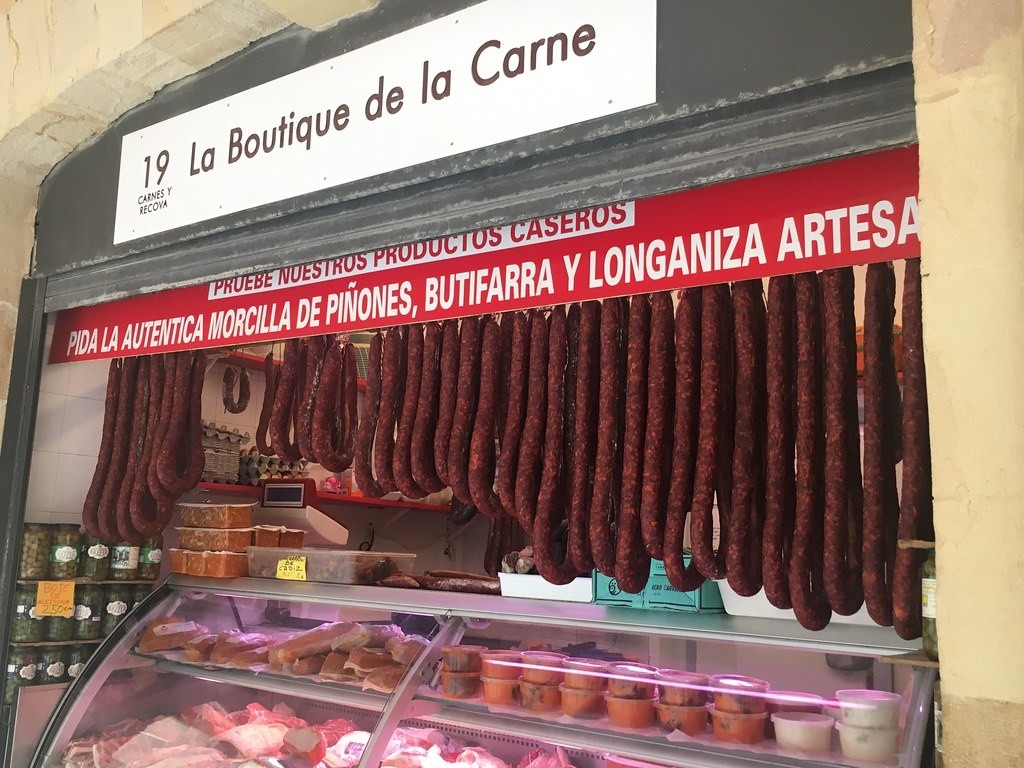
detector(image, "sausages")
[81,252,933,640]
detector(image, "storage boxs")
[497,572,593,602]
[243,545,417,583]
[593,555,727,614]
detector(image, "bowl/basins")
[439,644,903,761]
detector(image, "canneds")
[17,522,164,582]
[919,546,939,661]
[3,583,153,703]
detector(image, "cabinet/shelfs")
[191,348,451,532]
